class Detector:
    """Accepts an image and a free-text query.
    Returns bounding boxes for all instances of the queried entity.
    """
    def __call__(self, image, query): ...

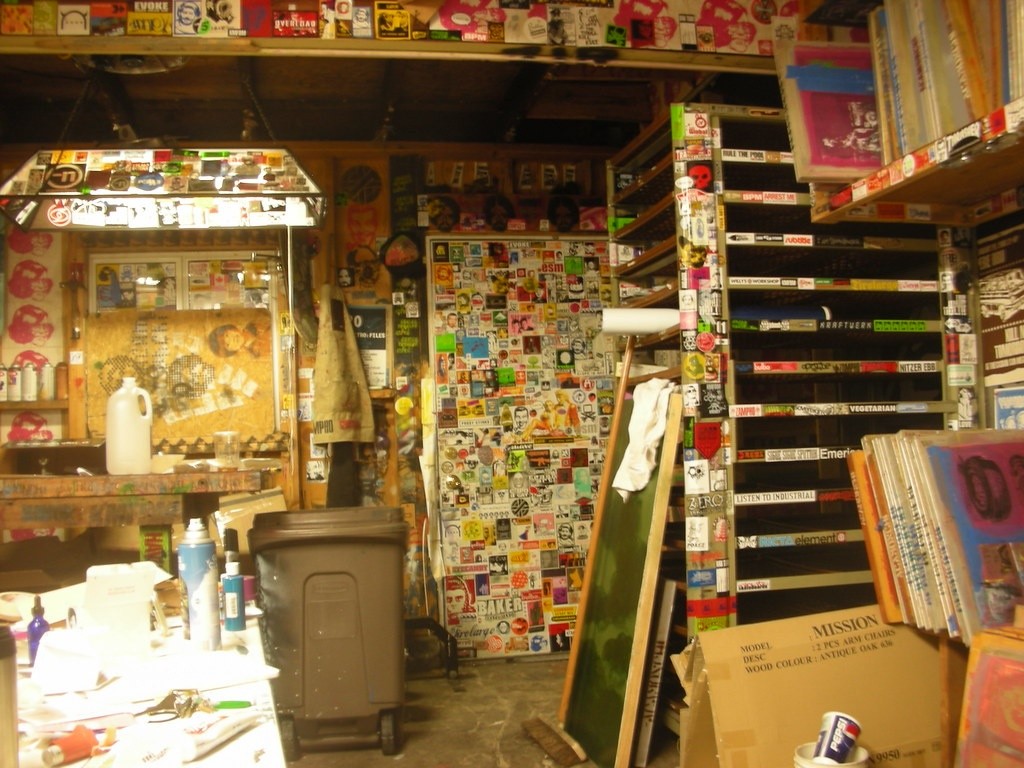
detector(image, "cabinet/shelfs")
[605,99,946,739]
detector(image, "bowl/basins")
[242,458,273,468]
[152,454,185,474]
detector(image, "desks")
[0,469,262,576]
[0,591,285,768]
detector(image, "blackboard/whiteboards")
[562,396,666,768]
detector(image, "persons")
[208,320,272,358]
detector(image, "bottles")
[56,362,68,400]
[176,517,221,650]
[7,364,21,401]
[27,595,50,665]
[39,362,55,400]
[0,364,7,402]
[22,363,37,401]
[222,562,246,632]
[106,377,153,475]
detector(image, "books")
[771,0,1024,183]
[847,428,1024,768]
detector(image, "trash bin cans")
[245,504,414,762]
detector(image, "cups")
[793,742,870,768]
[815,711,861,764]
[214,432,241,471]
